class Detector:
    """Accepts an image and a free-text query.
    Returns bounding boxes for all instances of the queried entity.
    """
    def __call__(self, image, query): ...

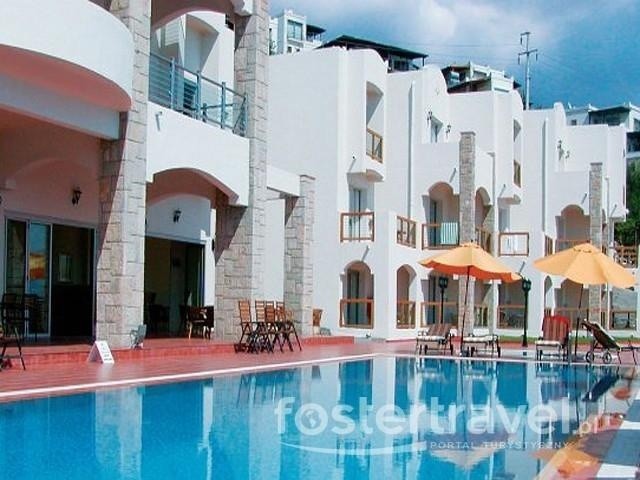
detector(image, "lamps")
[173,204,182,223]
[71,184,83,206]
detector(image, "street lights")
[517,275,532,348]
[455,359,479,462]
[436,273,450,346]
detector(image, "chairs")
[178,301,215,340]
[416,315,640,365]
[235,299,303,354]
[0,292,42,371]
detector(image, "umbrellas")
[415,239,523,354]
[531,242,638,355]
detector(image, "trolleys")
[578,322,617,363]
[581,365,625,405]
